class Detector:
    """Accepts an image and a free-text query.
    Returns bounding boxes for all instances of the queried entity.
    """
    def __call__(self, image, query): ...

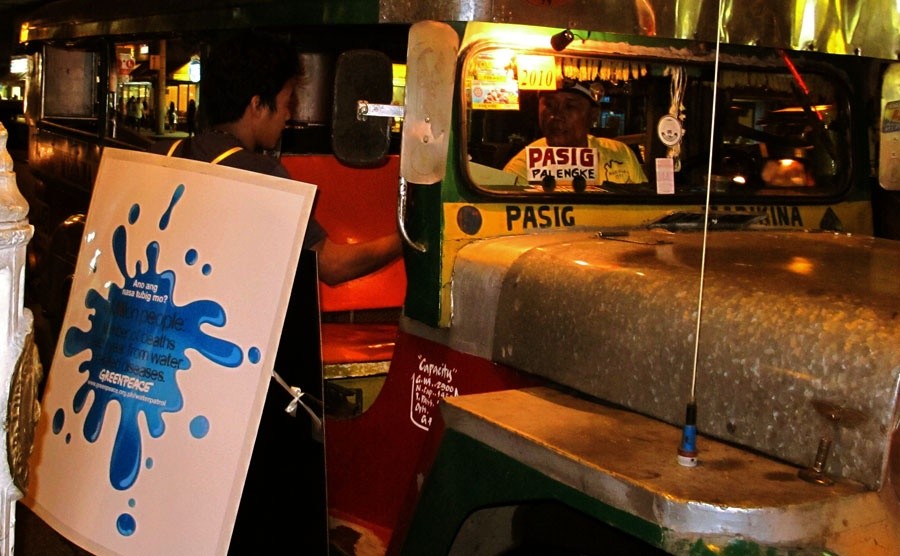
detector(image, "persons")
[166,101,178,132]
[146,27,403,285]
[186,99,195,136]
[118,96,149,132]
[501,78,650,186]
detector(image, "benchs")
[275,152,402,377]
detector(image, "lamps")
[551,27,586,52]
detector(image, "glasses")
[561,79,594,98]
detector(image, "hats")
[538,78,606,107]
